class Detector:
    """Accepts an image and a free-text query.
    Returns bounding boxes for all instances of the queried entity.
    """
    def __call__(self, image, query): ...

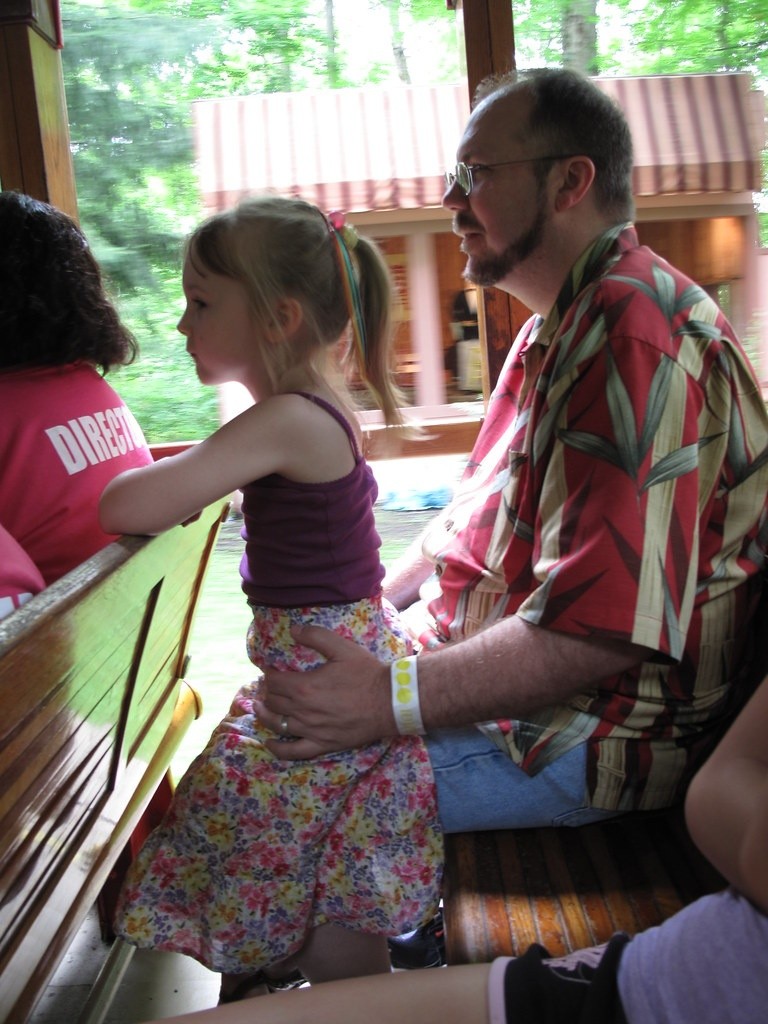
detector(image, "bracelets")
[390,656,428,736]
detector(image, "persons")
[0,190,153,578]
[0,525,46,622]
[462,279,479,340]
[153,674,768,1024]
[99,197,445,1001]
[250,69,768,989]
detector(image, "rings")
[281,716,292,738]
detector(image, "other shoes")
[386,912,445,970]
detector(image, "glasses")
[444,155,573,196]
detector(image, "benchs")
[0,488,237,1024]
[438,552,768,968]
